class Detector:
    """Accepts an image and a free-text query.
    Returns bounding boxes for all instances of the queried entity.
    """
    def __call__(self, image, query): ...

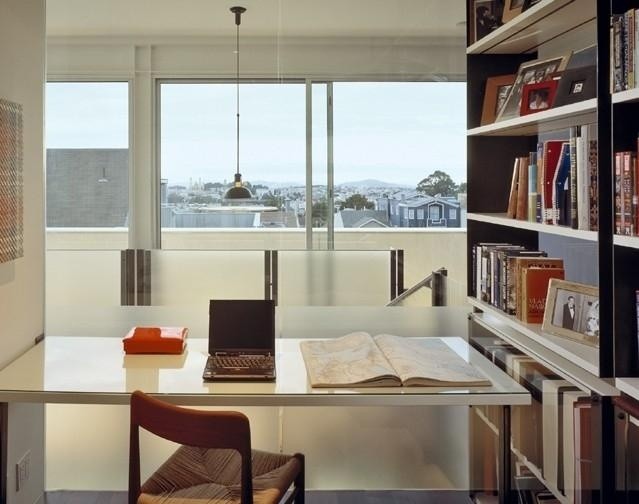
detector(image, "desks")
[1,336,531,504]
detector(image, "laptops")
[203,299,277,381]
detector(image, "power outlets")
[12,451,35,495]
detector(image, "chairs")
[128,391,306,503]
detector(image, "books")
[505,126,639,237]
[472,242,566,324]
[299,333,494,387]
[609,8,639,93]
[484,341,638,502]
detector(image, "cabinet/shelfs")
[466,1,639,504]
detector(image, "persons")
[476,4,496,38]
[563,295,576,329]
[530,95,548,107]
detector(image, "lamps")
[222,6,255,200]
